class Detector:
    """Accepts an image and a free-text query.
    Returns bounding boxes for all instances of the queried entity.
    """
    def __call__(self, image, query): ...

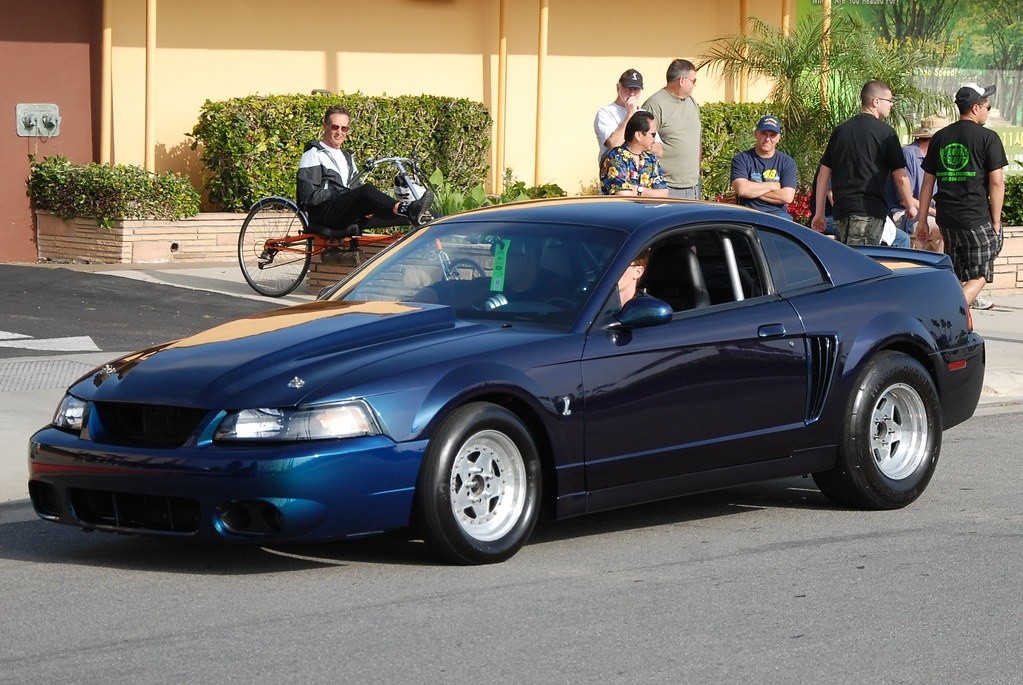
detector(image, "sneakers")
[407,190,434,227]
[971,295,995,310]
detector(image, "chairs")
[533,239,710,311]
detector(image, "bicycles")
[239,156,488,297]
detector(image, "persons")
[884,114,995,310]
[561,246,656,315]
[729,116,797,223]
[914,83,1010,308]
[641,59,701,199]
[599,110,669,199]
[594,69,665,163]
[810,80,917,246]
[806,160,910,248]
[295,104,435,230]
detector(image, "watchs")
[637,185,644,197]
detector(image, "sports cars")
[28,196,986,568]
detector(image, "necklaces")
[627,149,641,156]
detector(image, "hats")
[955,82,996,108]
[911,113,951,138]
[619,68,643,90]
[757,114,781,134]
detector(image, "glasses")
[638,129,656,138]
[871,95,893,104]
[326,123,350,132]
[970,103,992,111]
[677,75,696,85]
[600,260,639,270]
